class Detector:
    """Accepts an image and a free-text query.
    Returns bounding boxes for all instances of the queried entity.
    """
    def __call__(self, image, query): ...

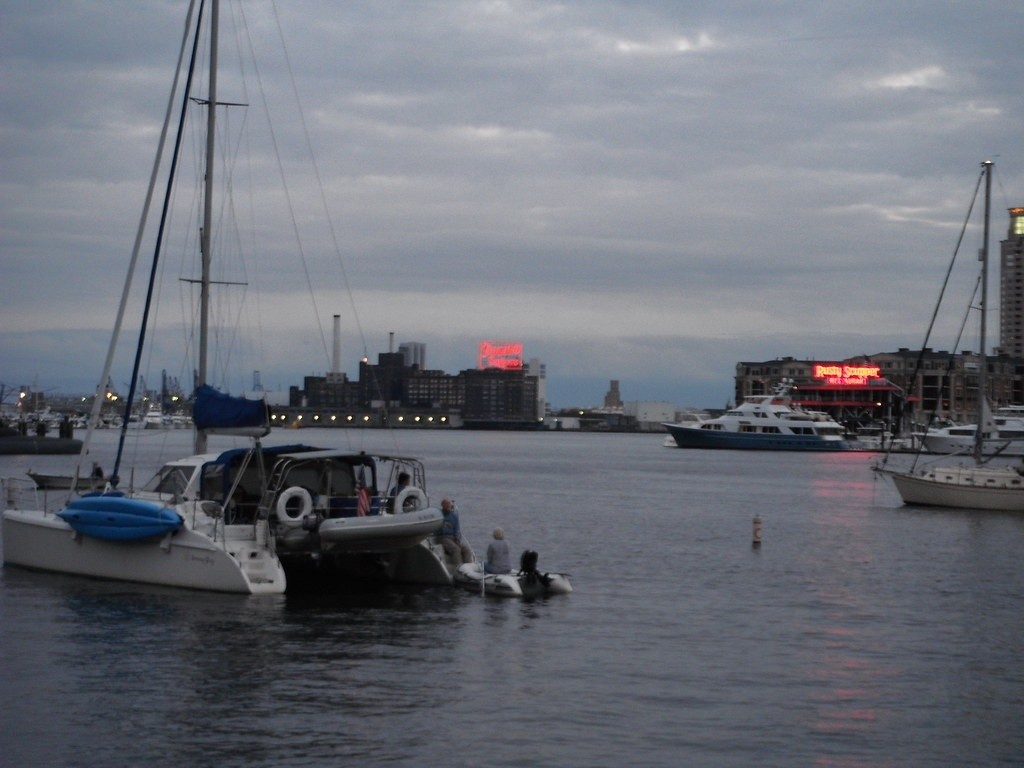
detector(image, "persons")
[93,467,104,492]
[437,499,472,565]
[386,472,417,514]
[484,527,512,573]
[105,476,119,494]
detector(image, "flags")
[356,465,371,516]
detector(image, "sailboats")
[870,159,1024,511]
[0,0,475,594]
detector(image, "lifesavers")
[277,485,313,527]
[394,486,428,515]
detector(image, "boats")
[458,560,573,596]
[911,405,1024,455]
[855,431,907,451]
[660,378,868,452]
[26,471,120,488]
[7,397,194,429]
